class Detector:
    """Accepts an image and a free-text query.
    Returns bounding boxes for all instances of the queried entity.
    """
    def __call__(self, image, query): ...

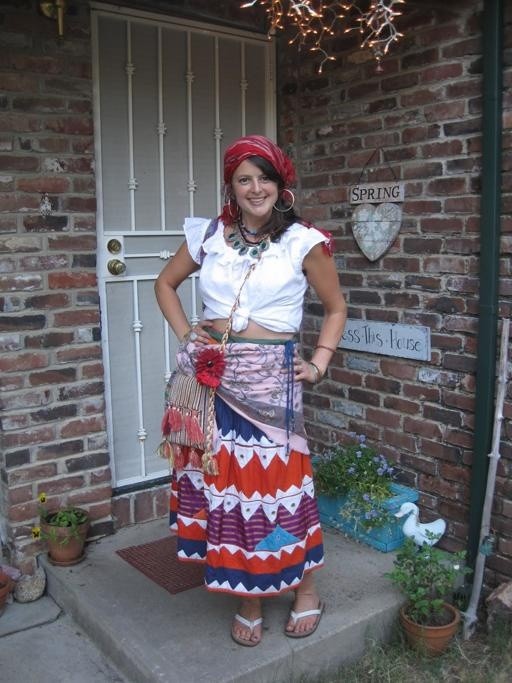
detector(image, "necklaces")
[228,217,280,258]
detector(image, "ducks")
[394,501,447,561]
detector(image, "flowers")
[310,430,405,549]
[381,529,474,627]
[195,348,227,388]
[31,492,87,555]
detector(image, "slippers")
[231,613,263,647]
[284,600,325,637]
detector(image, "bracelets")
[314,346,336,353]
[307,362,321,384]
[181,330,191,344]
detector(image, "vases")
[399,599,462,657]
[312,455,419,553]
[40,508,89,567]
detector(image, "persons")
[154,135,349,648]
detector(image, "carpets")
[115,536,207,595]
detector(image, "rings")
[190,333,198,341]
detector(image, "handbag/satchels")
[161,371,218,453]
